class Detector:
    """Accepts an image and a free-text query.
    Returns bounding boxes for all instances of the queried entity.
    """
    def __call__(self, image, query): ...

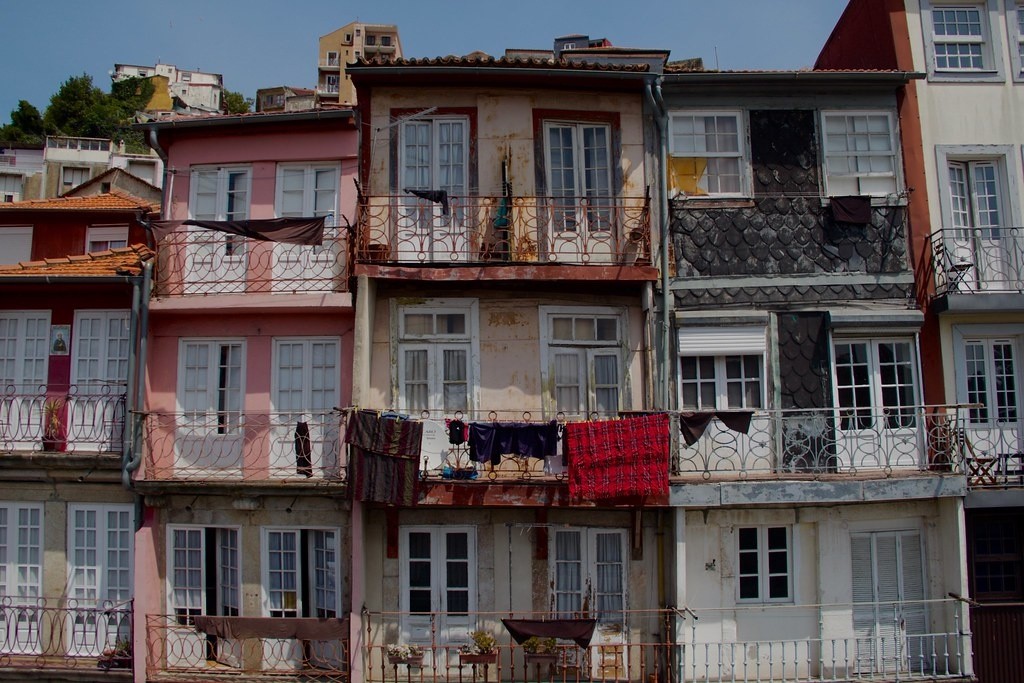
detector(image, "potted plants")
[523,637,560,663]
[98,636,131,668]
[387,644,406,663]
[41,397,63,452]
[459,630,498,663]
[407,644,424,665]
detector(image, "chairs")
[952,428,997,486]
[934,242,976,294]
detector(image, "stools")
[369,244,391,263]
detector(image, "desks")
[998,453,1024,490]
